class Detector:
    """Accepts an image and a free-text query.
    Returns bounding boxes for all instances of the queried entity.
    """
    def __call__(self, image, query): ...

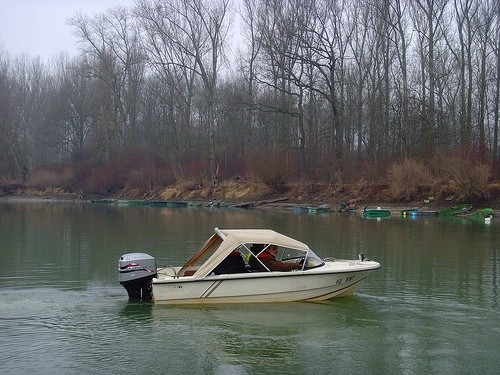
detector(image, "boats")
[119,227,381,304]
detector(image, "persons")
[248,243,265,271]
[255,243,303,271]
